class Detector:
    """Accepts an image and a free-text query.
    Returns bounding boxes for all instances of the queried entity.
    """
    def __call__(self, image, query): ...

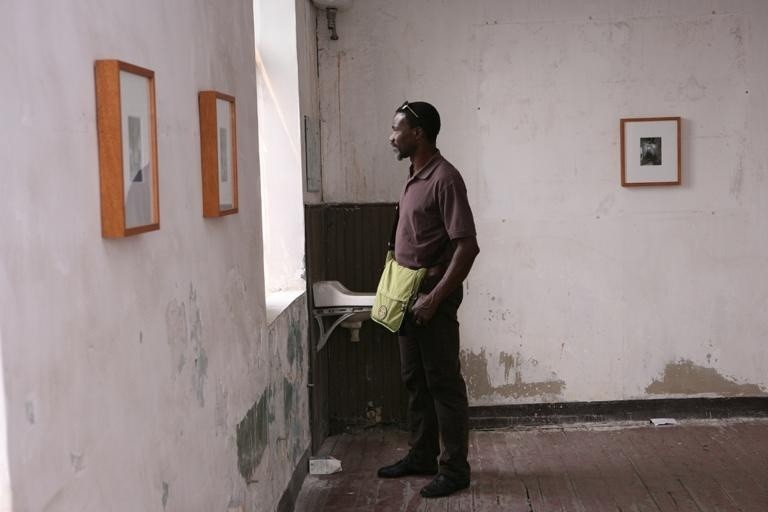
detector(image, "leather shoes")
[377,453,439,478]
[420,472,471,498]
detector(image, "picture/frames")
[95,57,159,240]
[199,89,241,221]
[619,115,680,189]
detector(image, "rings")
[415,318,423,325]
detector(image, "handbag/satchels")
[369,250,427,335]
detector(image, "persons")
[376,98,480,501]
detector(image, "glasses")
[400,100,420,120]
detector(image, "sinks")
[312,279,379,308]
